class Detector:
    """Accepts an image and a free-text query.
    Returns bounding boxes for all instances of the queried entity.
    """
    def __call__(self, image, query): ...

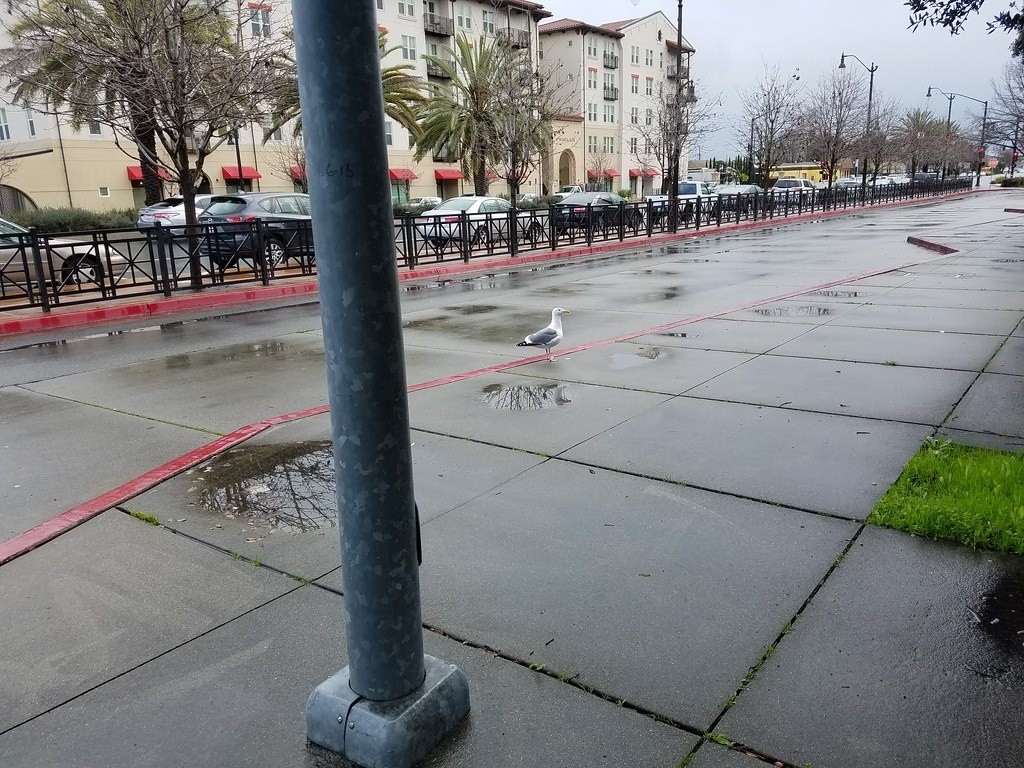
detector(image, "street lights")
[838,50,879,186]
[674,79,698,231]
[926,85,956,180]
[228,121,245,191]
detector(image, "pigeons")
[515,307,571,362]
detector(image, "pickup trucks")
[643,180,720,221]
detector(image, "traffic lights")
[979,145,986,159]
[1013,152,1019,164]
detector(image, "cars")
[813,171,987,193]
[770,178,817,206]
[420,197,543,244]
[0,217,127,285]
[137,193,220,235]
[716,185,770,212]
[402,196,442,207]
[509,194,538,202]
[548,192,644,232]
[198,191,314,266]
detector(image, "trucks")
[552,183,606,198]
